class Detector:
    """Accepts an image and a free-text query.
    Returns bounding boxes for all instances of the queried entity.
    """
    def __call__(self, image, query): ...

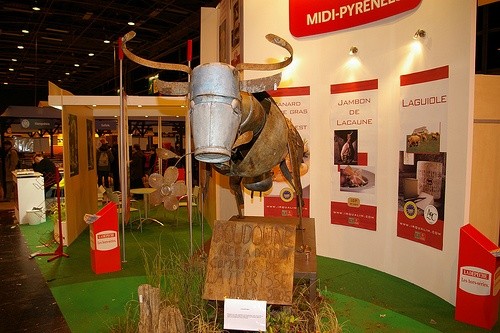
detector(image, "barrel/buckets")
[25,210,42,226]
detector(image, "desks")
[130,188,164,229]
[12,170,46,225]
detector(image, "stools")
[117,207,142,233]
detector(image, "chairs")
[176,185,201,228]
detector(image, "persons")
[3,141,19,202]
[32,154,62,200]
[96,139,156,201]
